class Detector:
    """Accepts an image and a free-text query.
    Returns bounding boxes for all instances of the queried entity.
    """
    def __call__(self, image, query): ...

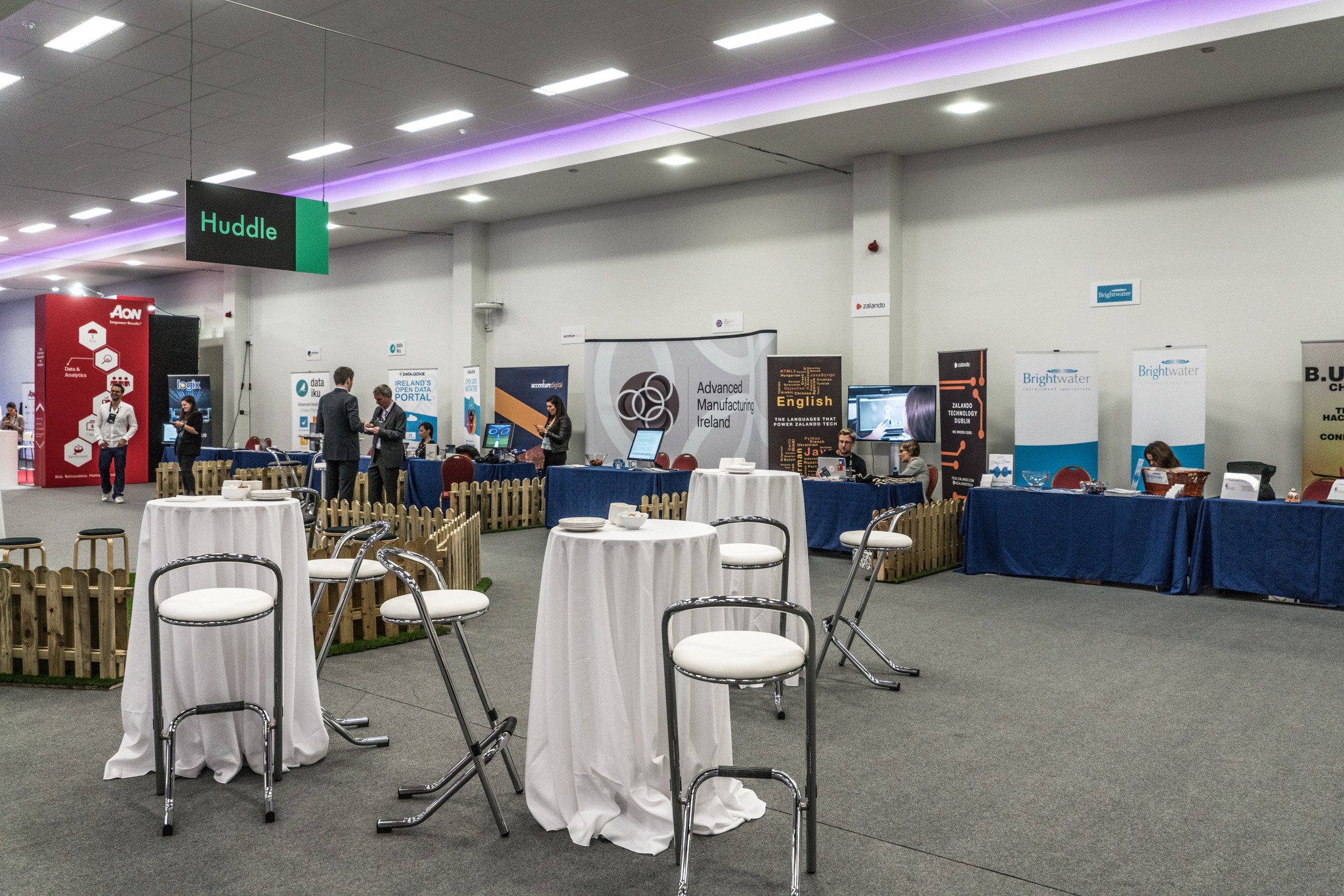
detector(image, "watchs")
[544,430,547,433]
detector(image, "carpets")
[18,471,33,484]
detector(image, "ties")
[378,410,387,448]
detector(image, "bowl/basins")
[1080,482,1109,494]
[221,486,252,501]
[741,462,756,467]
[618,512,649,530]
[1022,470,1050,489]
[585,454,607,467]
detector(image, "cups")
[234,443,239,449]
[719,458,747,472]
[223,480,262,499]
[255,445,261,451]
[406,451,411,457]
[608,502,637,527]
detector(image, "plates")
[558,517,606,532]
[724,465,755,475]
[250,490,292,501]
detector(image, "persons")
[862,422,887,440]
[0,402,25,456]
[902,385,935,442]
[411,422,439,459]
[535,395,572,479]
[93,383,138,504]
[171,395,203,497]
[363,384,407,516]
[1144,441,1182,468]
[816,428,867,478]
[316,366,365,539]
[899,440,930,495]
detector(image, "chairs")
[655,452,670,468]
[671,453,698,471]
[245,436,272,450]
[926,463,939,502]
[1299,476,1344,501]
[1051,465,1092,489]
[440,454,474,511]
[17,432,35,469]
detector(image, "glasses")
[838,440,853,445]
[899,449,912,453]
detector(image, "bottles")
[893,467,899,475]
[1288,488,1299,500]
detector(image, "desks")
[103,442,1344,855]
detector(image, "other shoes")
[184,490,198,496]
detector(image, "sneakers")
[115,496,124,503]
[102,488,112,501]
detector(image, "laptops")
[818,457,852,480]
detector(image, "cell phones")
[534,424,537,426]
[6,422,10,423]
[169,421,173,423]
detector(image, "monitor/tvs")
[846,385,937,444]
[856,393,908,439]
[309,422,320,452]
[627,427,666,471]
[482,423,515,455]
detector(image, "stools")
[305,452,325,512]
[370,540,525,836]
[145,553,285,839]
[0,537,47,570]
[663,594,820,896]
[307,519,393,745]
[814,502,920,690]
[73,528,130,585]
[268,447,304,500]
[282,487,321,549]
[710,515,791,720]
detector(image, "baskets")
[1141,467,1211,497]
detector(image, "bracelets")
[182,424,185,428]
